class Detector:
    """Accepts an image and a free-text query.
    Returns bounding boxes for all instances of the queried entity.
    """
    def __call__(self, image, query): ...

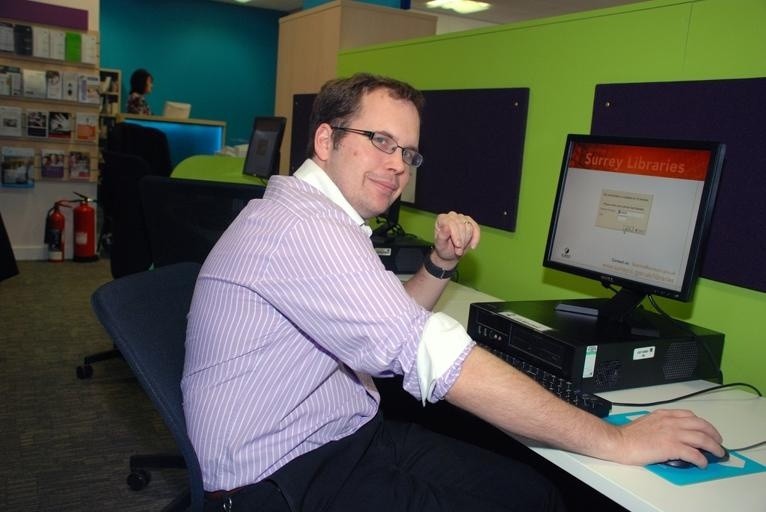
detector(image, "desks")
[377,273,765,512]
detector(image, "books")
[0,21,121,191]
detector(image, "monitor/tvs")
[164,101,190,119]
[242,116,289,179]
[542,132,727,336]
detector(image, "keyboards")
[479,338,612,419]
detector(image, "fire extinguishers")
[44,200,73,262]
[68,191,106,263]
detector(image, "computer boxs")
[371,235,434,275]
[469,298,726,393]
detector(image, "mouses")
[664,445,729,469]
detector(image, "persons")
[180,69,727,511]
[126,70,156,116]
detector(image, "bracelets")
[420,246,459,281]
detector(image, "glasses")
[331,126,423,168]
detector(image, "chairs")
[74,175,269,380]
[89,263,212,509]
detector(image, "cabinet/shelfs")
[100,69,122,132]
[0,18,100,183]
[272,1,437,176]
